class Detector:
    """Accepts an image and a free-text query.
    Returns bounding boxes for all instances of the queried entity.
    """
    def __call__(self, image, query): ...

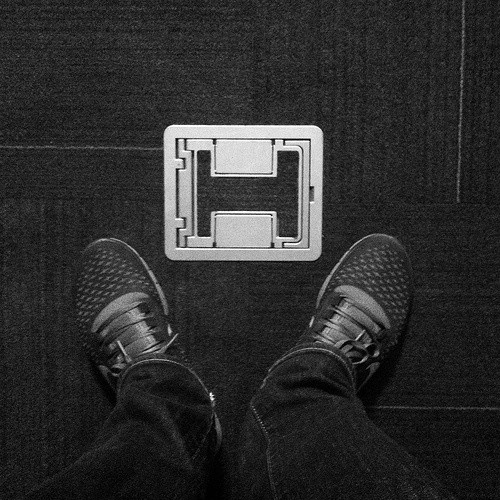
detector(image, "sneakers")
[295,234,413,394]
[72,239,222,457]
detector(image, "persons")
[21,233,462,500]
